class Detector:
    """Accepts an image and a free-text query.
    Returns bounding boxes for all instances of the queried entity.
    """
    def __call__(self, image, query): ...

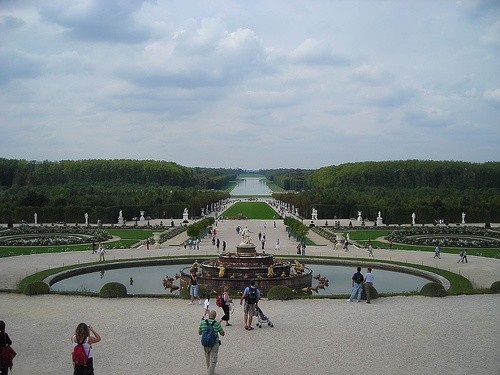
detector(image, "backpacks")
[355,273,363,284]
[215,293,228,307]
[244,286,258,304]
[72,336,92,366]
[201,319,218,348]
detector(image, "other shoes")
[188,302,194,304]
[202,318,204,320]
[226,324,232,326]
[248,327,254,331]
[198,299,202,305]
[347,299,352,302]
[244,325,248,330]
[366,301,370,304]
[356,299,360,303]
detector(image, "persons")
[240,281,260,330]
[146,241,150,249]
[198,310,225,375]
[184,238,200,250]
[0,320,12,375]
[219,286,233,326]
[202,295,210,320]
[91,240,106,262]
[71,323,101,375]
[187,271,201,305]
[236,226,242,234]
[433,245,441,259]
[242,226,254,244]
[208,221,226,252]
[257,221,392,256]
[347,266,374,304]
[456,249,468,263]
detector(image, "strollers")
[253,304,274,328]
